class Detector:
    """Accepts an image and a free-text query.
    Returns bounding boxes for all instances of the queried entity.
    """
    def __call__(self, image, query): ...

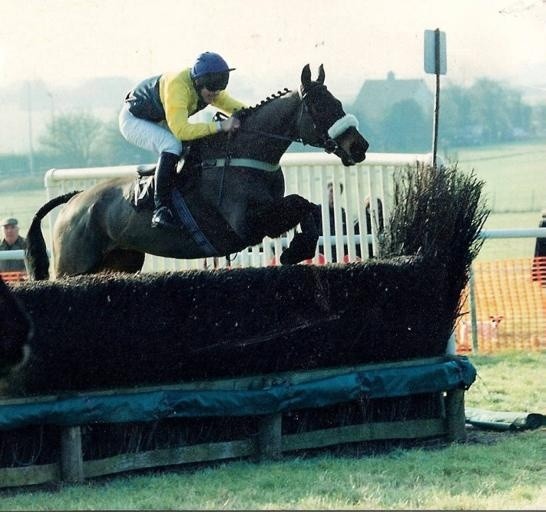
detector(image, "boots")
[151,152,181,230]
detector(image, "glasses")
[205,79,228,91]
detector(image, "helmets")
[191,52,236,77]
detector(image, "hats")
[2,218,18,225]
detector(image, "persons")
[349,196,384,259]
[117,51,251,231]
[0,218,24,250]
[313,181,352,265]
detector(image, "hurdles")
[1,260,477,488]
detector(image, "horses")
[22,63,369,284]
